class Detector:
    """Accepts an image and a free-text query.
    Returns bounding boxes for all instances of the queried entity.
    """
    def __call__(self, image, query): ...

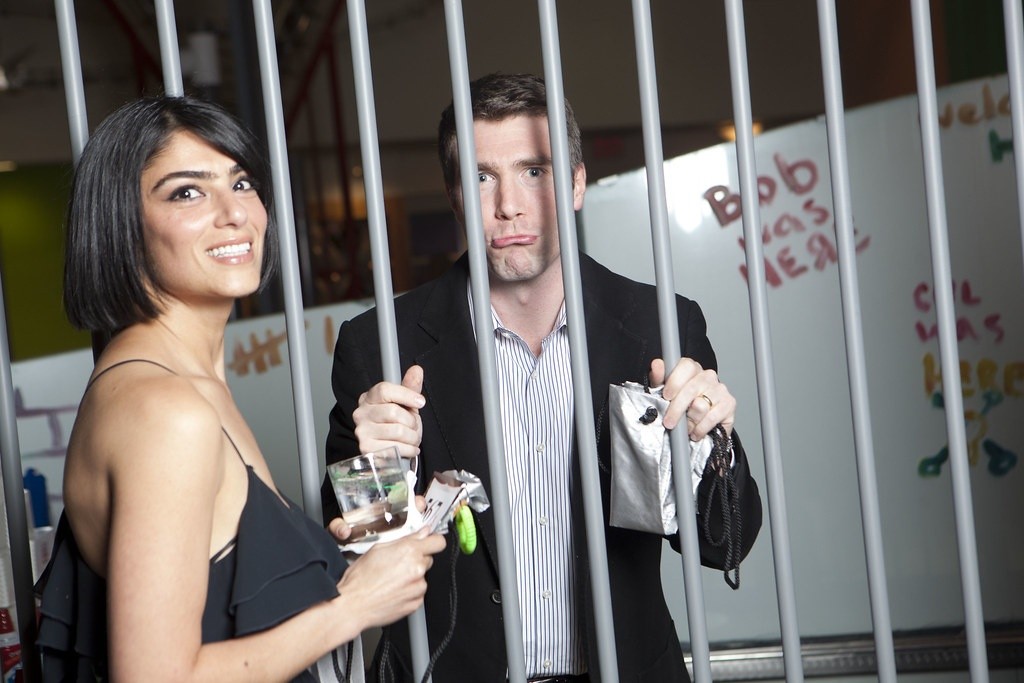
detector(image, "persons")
[320,73,762,683]
[29,94,447,683]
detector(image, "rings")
[698,393,713,406]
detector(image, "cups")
[327,447,419,546]
